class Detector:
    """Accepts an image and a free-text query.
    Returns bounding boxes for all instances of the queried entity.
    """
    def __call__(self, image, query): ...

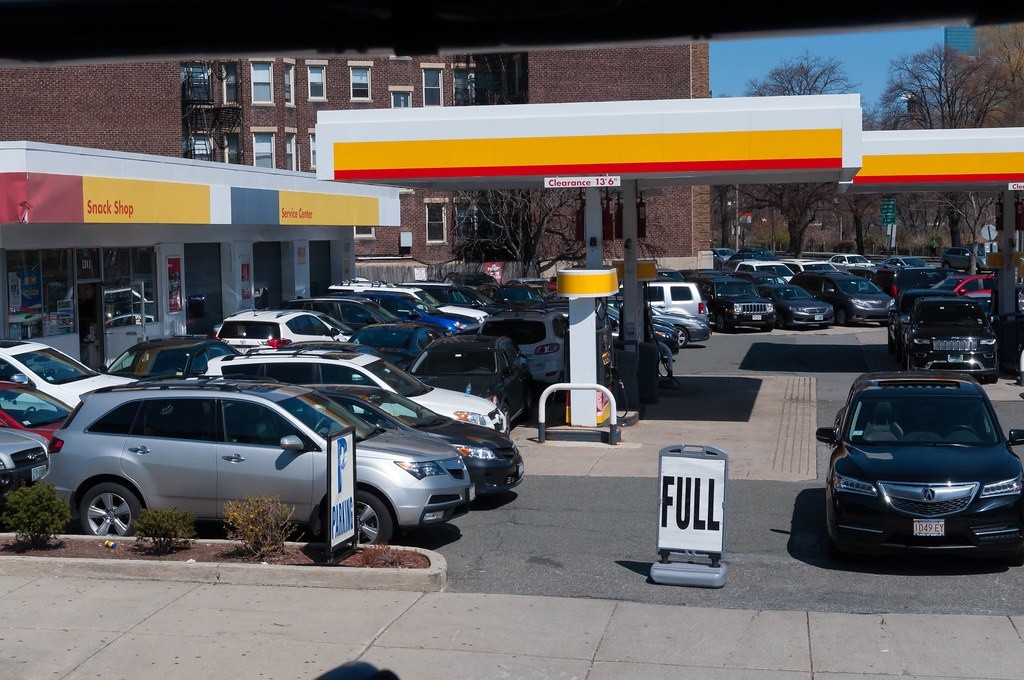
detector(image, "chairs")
[863,401,903,443]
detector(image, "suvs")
[40,374,477,546]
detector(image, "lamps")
[18,201,33,224]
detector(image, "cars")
[815,370,1024,556]
[940,245,992,275]
[0,247,1024,496]
